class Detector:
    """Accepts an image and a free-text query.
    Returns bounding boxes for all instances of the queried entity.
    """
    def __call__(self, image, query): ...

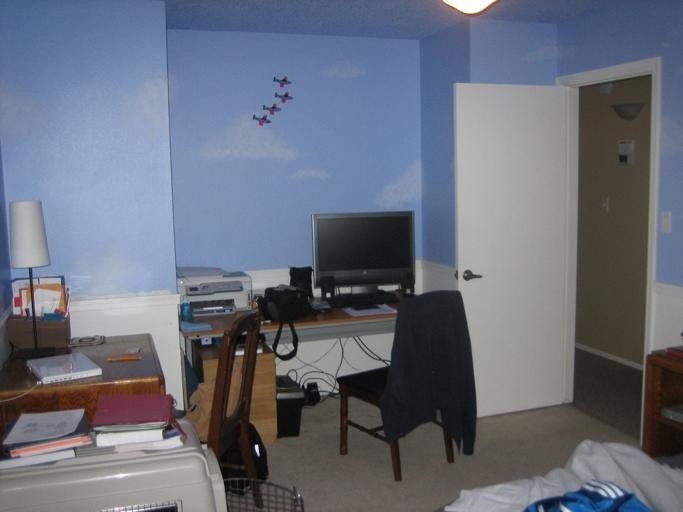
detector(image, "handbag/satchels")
[256,282,317,323]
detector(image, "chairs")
[335,290,476,480]
[198,307,262,507]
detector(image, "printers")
[176,265,253,325]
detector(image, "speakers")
[290,267,311,295]
[401,272,414,295]
[320,276,335,299]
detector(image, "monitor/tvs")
[311,211,420,286]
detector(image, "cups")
[181,303,191,320]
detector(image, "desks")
[180,296,399,447]
[1,331,163,427]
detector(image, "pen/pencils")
[107,357,142,361]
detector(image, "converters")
[308,382,321,402]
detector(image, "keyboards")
[324,291,402,308]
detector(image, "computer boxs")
[276,374,303,438]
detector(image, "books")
[26,283,67,322]
[0,393,186,471]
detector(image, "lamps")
[6,199,68,358]
[441,0,502,17]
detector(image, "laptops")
[27,352,104,386]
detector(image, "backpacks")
[222,414,271,495]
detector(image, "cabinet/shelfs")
[642,346,683,466]
